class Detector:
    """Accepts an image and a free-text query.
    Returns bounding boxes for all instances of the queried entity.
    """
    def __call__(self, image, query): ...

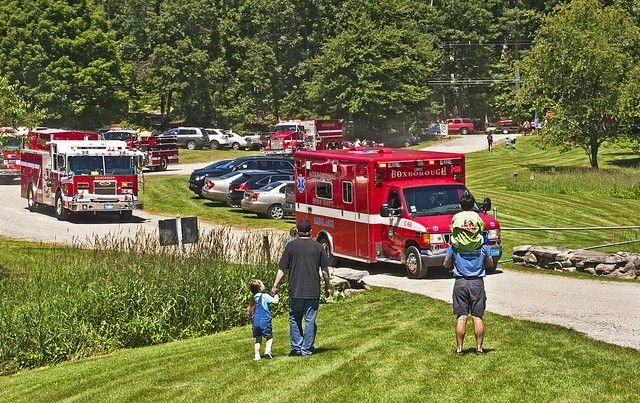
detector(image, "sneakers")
[476,348,485,354]
[254,354,262,360]
[289,348,301,355]
[264,350,272,358]
[456,349,464,355]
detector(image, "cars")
[240,179,295,219]
[201,169,267,208]
[485,123,501,133]
[195,160,234,171]
[262,132,277,145]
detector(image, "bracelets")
[273,283,278,288]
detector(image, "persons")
[246,279,279,360]
[505,137,510,149]
[451,194,485,251]
[486,133,493,151]
[443,239,494,355]
[428,195,436,208]
[272,220,331,356]
[511,135,516,149]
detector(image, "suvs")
[205,128,230,149]
[496,119,523,133]
[188,157,295,201]
[443,118,474,135]
[166,126,209,151]
[225,171,295,210]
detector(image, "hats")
[297,219,312,232]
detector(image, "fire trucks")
[104,128,179,171]
[0,126,102,181]
[19,126,139,221]
[291,140,502,280]
[265,118,343,157]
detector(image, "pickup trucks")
[225,129,262,150]
